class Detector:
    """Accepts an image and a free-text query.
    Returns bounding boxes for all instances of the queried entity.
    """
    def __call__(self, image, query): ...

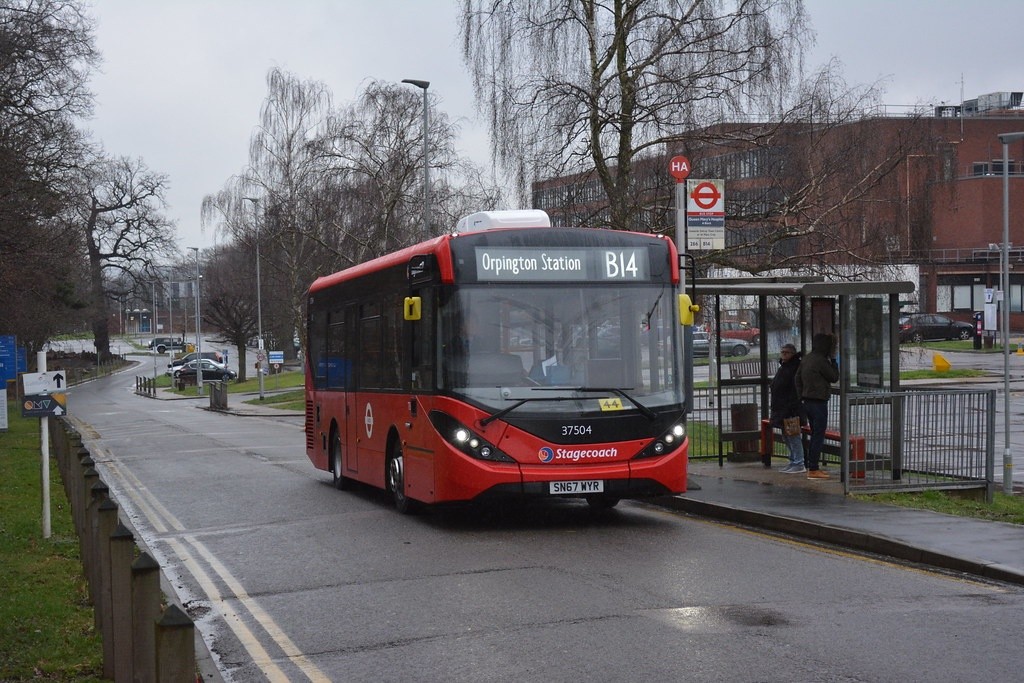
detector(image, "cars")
[698,320,761,346]
[658,331,751,357]
[165,358,229,379]
[899,314,974,343]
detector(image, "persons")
[794,333,839,480]
[442,315,484,372]
[770,344,807,474]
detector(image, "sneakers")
[786,464,806,474]
[779,464,794,472]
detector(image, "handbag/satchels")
[782,416,801,436]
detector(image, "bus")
[302,208,700,521]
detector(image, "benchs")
[761,418,865,479]
[728,359,781,379]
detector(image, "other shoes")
[807,470,829,479]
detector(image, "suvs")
[166,351,224,368]
[147,336,195,354]
[178,362,237,384]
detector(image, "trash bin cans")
[983,335,994,349]
[208,382,229,410]
[730,403,759,452]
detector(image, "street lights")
[187,247,203,392]
[400,78,431,242]
[240,196,266,400]
[995,131,1024,498]
